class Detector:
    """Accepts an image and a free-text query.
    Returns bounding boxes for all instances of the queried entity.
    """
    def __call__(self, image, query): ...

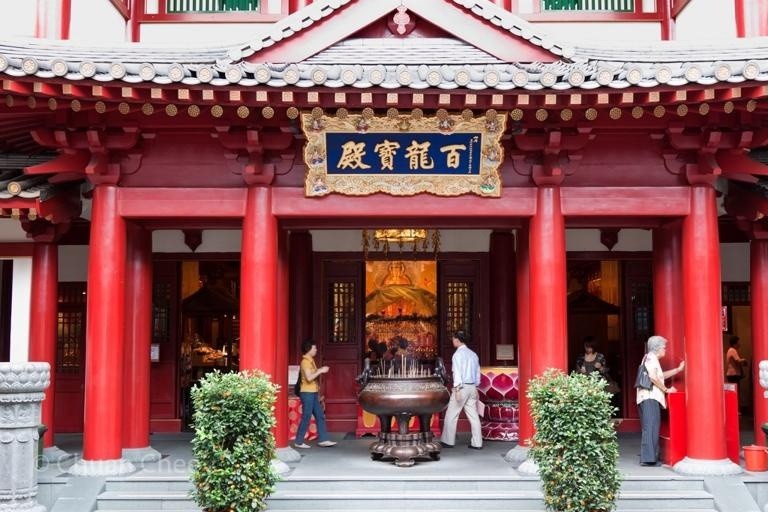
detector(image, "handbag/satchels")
[295,373,300,397]
[633,366,651,389]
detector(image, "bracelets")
[664,388,667,393]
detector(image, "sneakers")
[294,443,311,449]
[318,441,336,448]
[640,462,660,467]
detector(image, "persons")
[576,339,609,376]
[726,336,747,417]
[439,328,483,450]
[636,335,686,465]
[293,340,337,449]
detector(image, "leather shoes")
[440,442,452,448]
[467,445,480,450]
